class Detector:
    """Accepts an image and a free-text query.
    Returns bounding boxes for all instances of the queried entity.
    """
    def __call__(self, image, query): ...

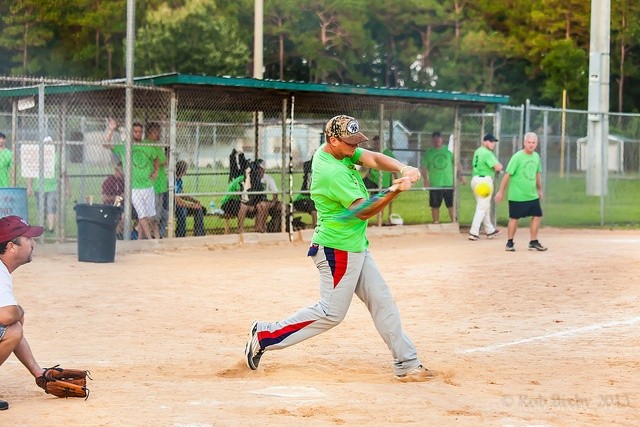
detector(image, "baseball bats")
[335,182,400,220]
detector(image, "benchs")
[172,209,236,234]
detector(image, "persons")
[0,132,13,189]
[244,114,440,382]
[100,114,206,240]
[362,135,399,196]
[0,215,48,410]
[492,132,548,251]
[221,159,317,235]
[419,131,468,224]
[468,134,504,240]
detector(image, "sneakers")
[395,365,437,381]
[505,242,515,251]
[469,234,480,240]
[529,243,547,251]
[245,319,267,370]
[487,229,501,238]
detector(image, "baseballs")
[475,181,492,199]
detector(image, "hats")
[325,115,370,144]
[0,215,44,242]
[483,134,498,141]
[432,131,441,136]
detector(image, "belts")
[479,174,486,177]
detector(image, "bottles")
[209,200,216,214]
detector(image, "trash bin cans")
[73,204,123,263]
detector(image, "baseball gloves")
[35,364,93,401]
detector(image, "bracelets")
[400,161,415,178]
